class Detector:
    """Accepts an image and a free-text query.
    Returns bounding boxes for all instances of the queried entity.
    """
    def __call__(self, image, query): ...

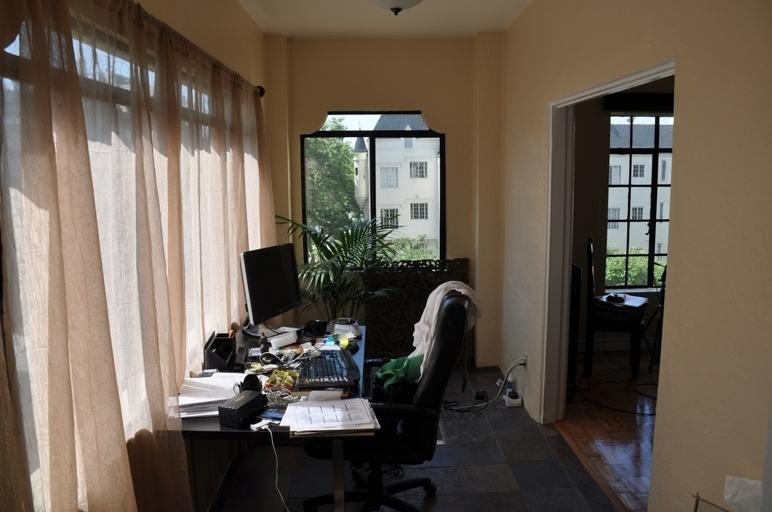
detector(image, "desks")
[156,320,368,512]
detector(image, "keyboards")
[293,347,361,391]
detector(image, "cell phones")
[249,415,271,432]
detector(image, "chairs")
[304,287,467,511]
[582,237,646,385]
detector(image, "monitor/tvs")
[240,242,303,338]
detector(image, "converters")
[475,391,488,401]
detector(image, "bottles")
[506,372,514,396]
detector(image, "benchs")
[366,257,471,393]
